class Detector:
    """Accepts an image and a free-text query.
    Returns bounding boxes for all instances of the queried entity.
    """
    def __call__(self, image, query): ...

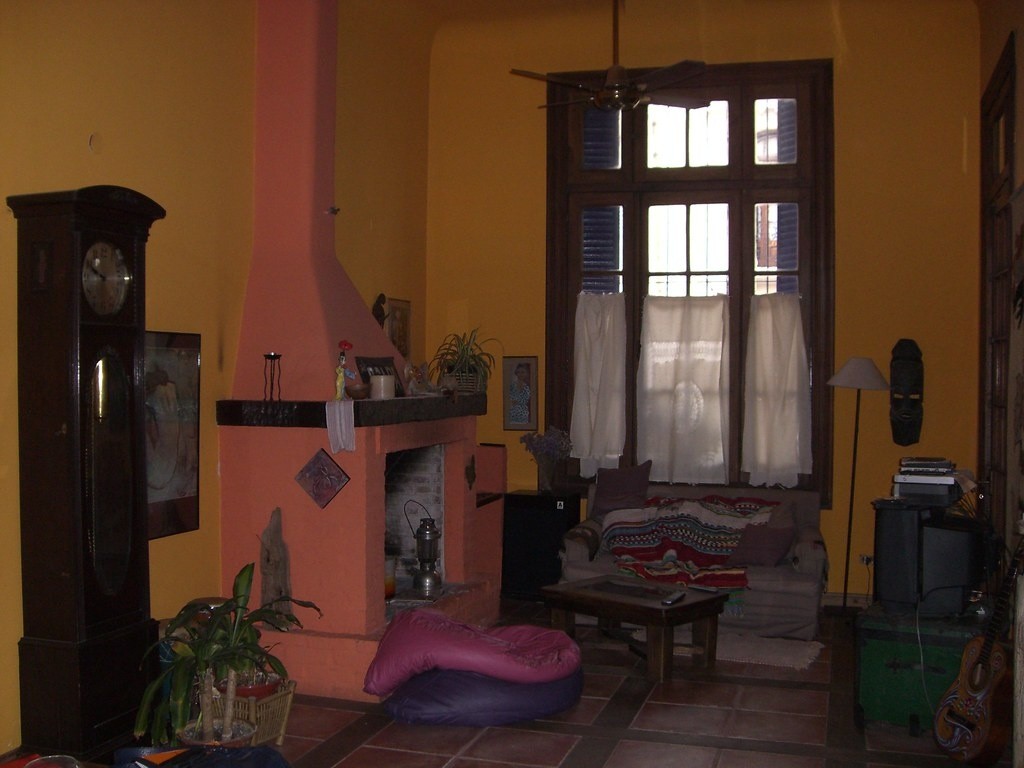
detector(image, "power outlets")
[859,553,873,565]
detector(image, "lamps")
[826,357,890,616]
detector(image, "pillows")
[731,523,794,568]
[590,460,652,518]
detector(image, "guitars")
[933,533,1023,768]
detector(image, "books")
[889,455,954,497]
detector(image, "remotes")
[688,584,719,593]
[661,591,685,606]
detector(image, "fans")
[508,0,711,113]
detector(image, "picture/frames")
[387,297,411,359]
[502,356,539,431]
[145,332,201,540]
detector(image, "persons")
[508,362,531,425]
[334,352,356,400]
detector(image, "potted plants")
[426,326,506,395]
[133,561,324,748]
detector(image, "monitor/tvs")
[872,507,990,619]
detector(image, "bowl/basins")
[345,384,370,400]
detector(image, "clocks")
[6,185,165,764]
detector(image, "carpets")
[631,626,824,672]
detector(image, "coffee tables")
[538,574,736,684]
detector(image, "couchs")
[579,484,830,642]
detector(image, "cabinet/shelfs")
[842,603,976,738]
